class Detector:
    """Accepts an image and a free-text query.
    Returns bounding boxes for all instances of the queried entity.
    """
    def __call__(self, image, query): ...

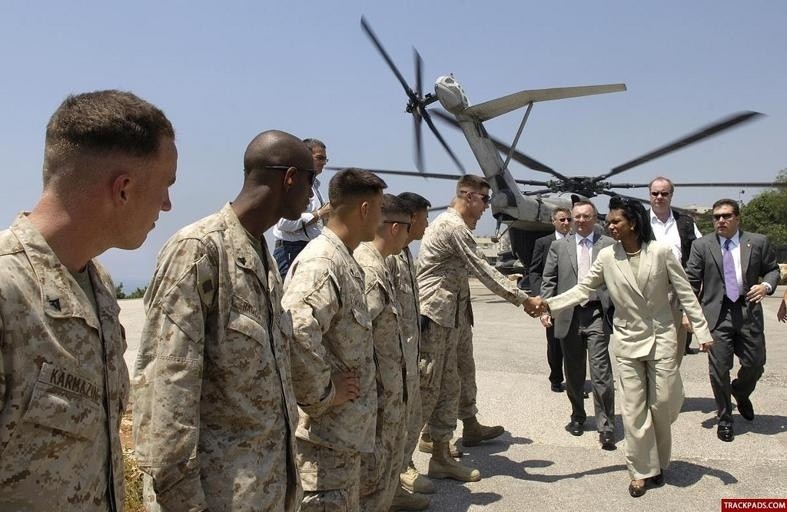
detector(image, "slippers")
[418,432,463,458]
[400,461,435,494]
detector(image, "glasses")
[384,219,412,233]
[574,214,593,222]
[461,190,490,203]
[559,217,571,223]
[264,164,317,186]
[652,191,669,197]
[314,155,330,163]
[710,212,733,221]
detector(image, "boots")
[427,441,481,482]
[389,483,431,511]
[461,414,505,447]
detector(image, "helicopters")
[322,16,787,291]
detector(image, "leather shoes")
[629,479,648,497]
[717,424,734,442]
[550,382,564,392]
[570,420,584,435]
[599,430,616,448]
[730,378,755,421]
[584,391,589,397]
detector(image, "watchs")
[761,284,772,294]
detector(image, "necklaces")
[624,247,643,256]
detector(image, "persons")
[281,168,388,511]
[390,192,430,512]
[539,199,618,448]
[272,139,330,286]
[399,173,543,494]
[409,512,413,512]
[1,90,178,512]
[684,199,781,407]
[530,197,714,498]
[419,196,505,458]
[530,207,590,398]
[354,194,413,511]
[648,177,702,355]
[133,131,316,512]
[777,286,787,324]
